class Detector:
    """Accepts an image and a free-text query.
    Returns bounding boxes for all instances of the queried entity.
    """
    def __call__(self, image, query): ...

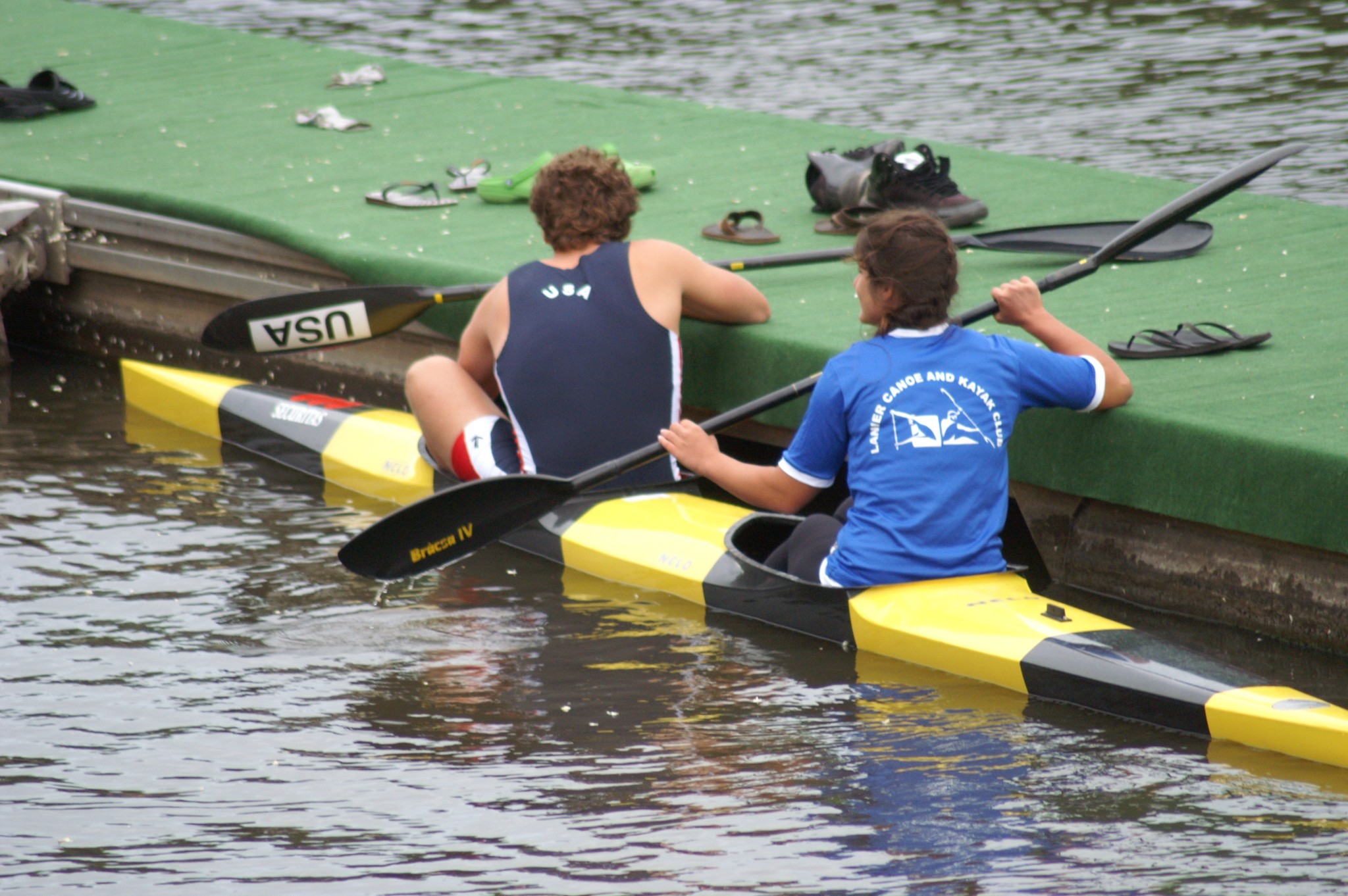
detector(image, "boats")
[120,356,1348,769]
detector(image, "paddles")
[198,219,1214,357]
[335,141,1311,580]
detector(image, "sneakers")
[806,137,905,211]
[865,144,988,229]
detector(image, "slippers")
[477,154,555,203]
[702,209,780,244]
[597,143,657,189]
[0,70,95,119]
[365,182,458,209]
[815,207,882,234]
[1152,321,1271,349]
[446,158,491,192]
[1108,329,1231,358]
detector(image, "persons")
[655,210,1134,588]
[405,148,773,491]
